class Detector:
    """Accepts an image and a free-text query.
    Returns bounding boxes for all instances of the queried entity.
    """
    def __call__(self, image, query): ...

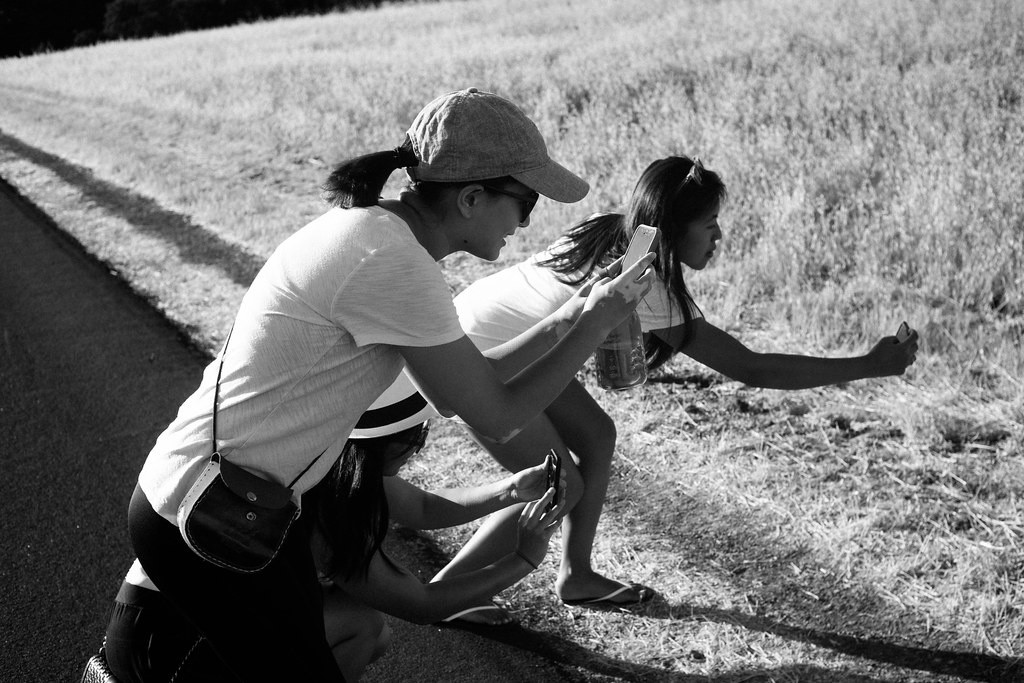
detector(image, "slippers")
[561,580,656,607]
[438,602,522,634]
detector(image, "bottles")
[597,310,645,390]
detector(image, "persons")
[128,88,656,682]
[104,380,566,683]
[426,153,918,627]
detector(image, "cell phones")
[616,224,663,282]
[544,449,562,512]
[895,321,911,343]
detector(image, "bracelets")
[516,550,538,571]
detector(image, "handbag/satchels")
[174,451,303,575]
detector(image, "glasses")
[486,184,539,223]
[675,159,705,192]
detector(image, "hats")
[405,88,590,203]
